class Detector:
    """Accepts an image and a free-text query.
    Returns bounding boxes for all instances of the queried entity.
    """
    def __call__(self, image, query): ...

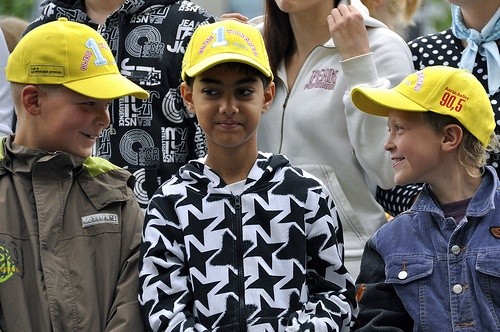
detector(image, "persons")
[350,65,500,332]
[220,0,415,284]
[0,17,150,331]
[1,16,15,139]
[11,0,219,215]
[137,20,359,331]
[375,0,500,217]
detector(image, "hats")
[181,20,274,83]
[3,17,150,100]
[350,66,496,150]
